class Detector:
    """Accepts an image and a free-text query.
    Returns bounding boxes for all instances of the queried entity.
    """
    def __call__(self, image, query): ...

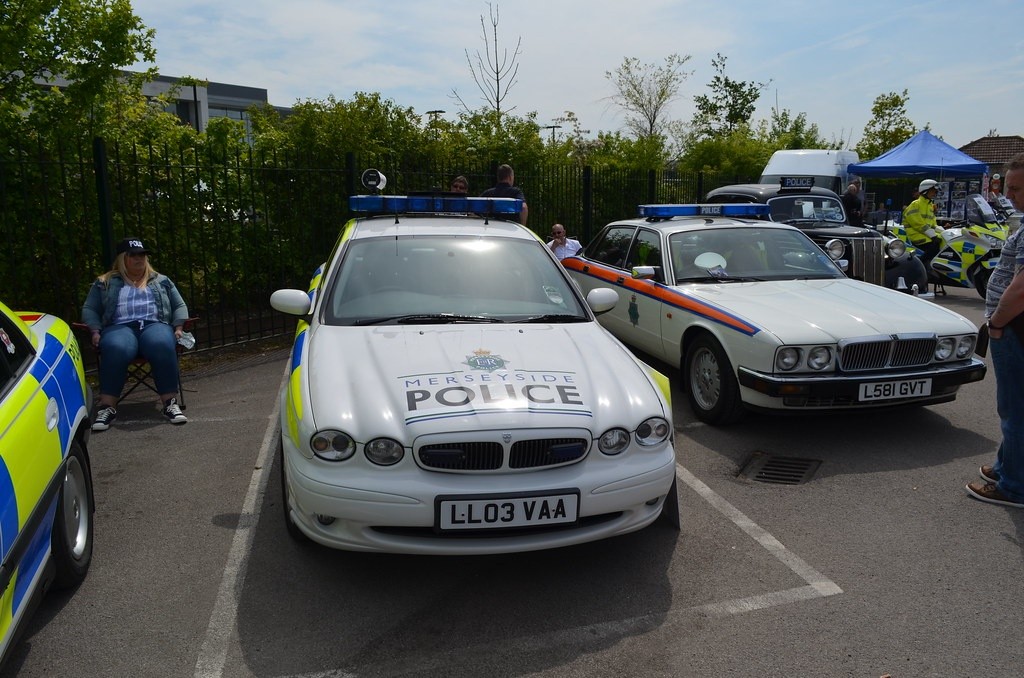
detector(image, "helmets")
[919,179,941,194]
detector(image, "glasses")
[451,184,467,190]
[553,231,561,235]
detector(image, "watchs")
[986,319,1003,331]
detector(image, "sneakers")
[966,482,1024,508]
[980,464,1000,482]
[93,405,116,430]
[163,397,187,423]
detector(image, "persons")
[83,239,187,430]
[852,180,868,216]
[450,176,468,193]
[479,164,528,227]
[843,184,865,227]
[966,152,1024,509]
[546,224,583,262]
[903,179,942,272]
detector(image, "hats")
[116,239,152,253]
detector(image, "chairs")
[73,316,198,412]
[727,243,767,274]
[865,191,876,212]
[645,242,683,282]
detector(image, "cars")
[558,202,987,430]
[0,295,99,678]
[268,192,683,565]
[706,176,936,301]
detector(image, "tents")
[845,130,988,226]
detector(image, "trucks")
[758,147,863,196]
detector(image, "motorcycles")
[863,193,1018,299]
